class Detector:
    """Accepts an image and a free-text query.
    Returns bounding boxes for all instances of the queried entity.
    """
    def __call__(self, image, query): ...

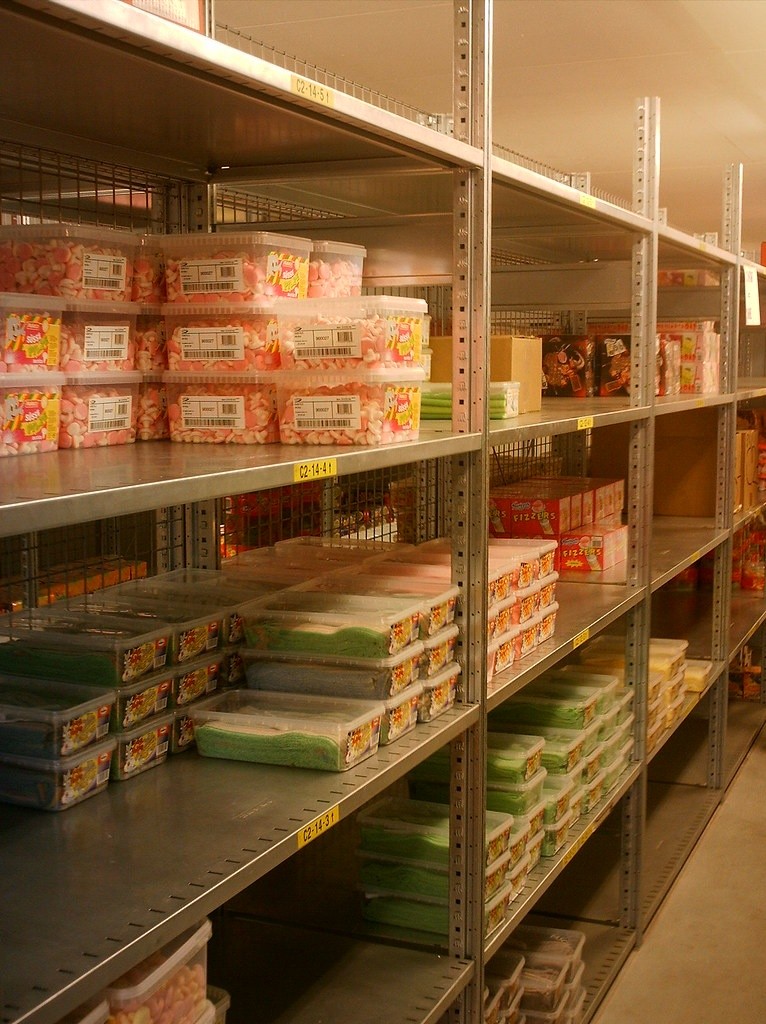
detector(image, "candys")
[0,243,422,452]
[105,954,206,1024]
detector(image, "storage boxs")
[540,334,697,397]
[353,635,714,939]
[429,336,541,413]
[483,925,587,1024]
[58,917,229,1024]
[490,475,628,571]
[1,537,558,810]
[734,430,760,518]
[421,382,521,419]
[1,225,433,457]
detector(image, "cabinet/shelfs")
[1,0,474,1024]
[645,163,766,794]
[493,96,734,967]
[185,1,650,1024]
